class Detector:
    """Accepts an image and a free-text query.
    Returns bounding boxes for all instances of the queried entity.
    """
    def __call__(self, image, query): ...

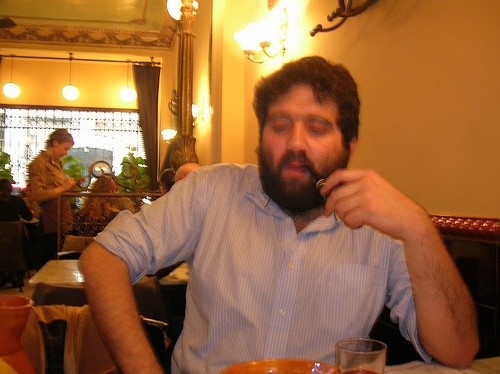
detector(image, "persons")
[0,129,202,288]
[78,56,479,374]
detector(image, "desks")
[27,259,189,337]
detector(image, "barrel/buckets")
[0,296,35,357]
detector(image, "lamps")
[62,52,80,100]
[3,55,21,98]
[118,60,137,102]
[236,8,288,63]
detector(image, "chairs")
[17,305,176,374]
[0,221,29,292]
[62,234,96,252]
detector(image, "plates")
[219,358,336,374]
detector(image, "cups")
[335,337,387,374]
